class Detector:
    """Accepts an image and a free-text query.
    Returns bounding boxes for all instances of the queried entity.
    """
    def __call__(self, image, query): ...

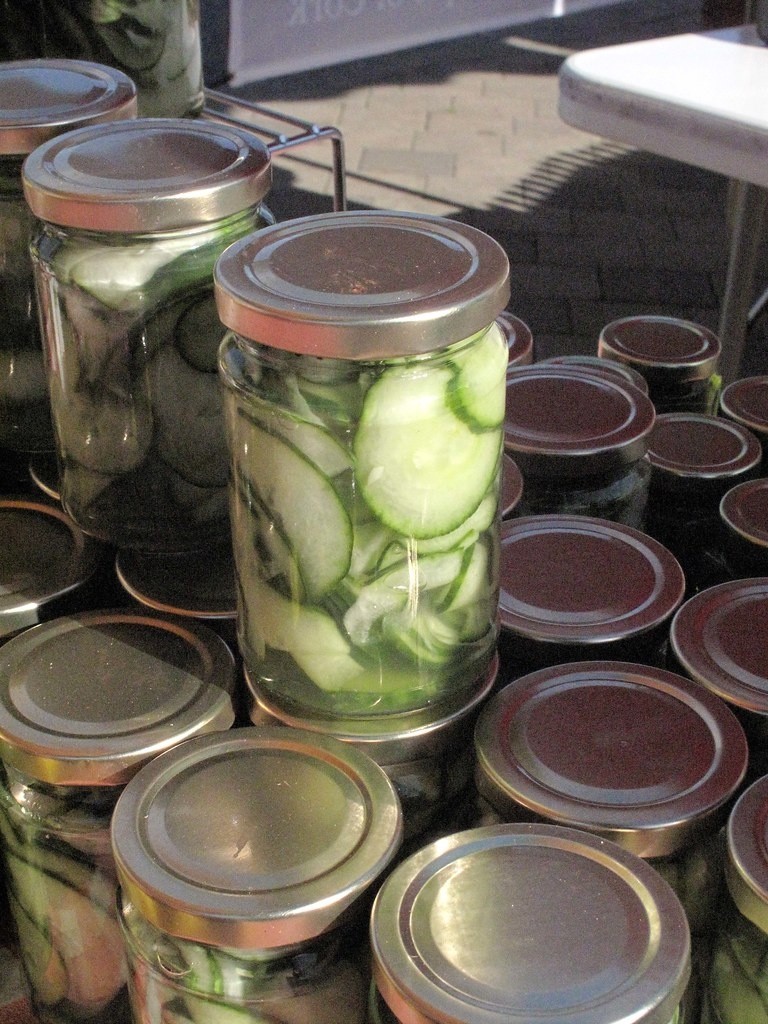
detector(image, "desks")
[558,22,768,390]
